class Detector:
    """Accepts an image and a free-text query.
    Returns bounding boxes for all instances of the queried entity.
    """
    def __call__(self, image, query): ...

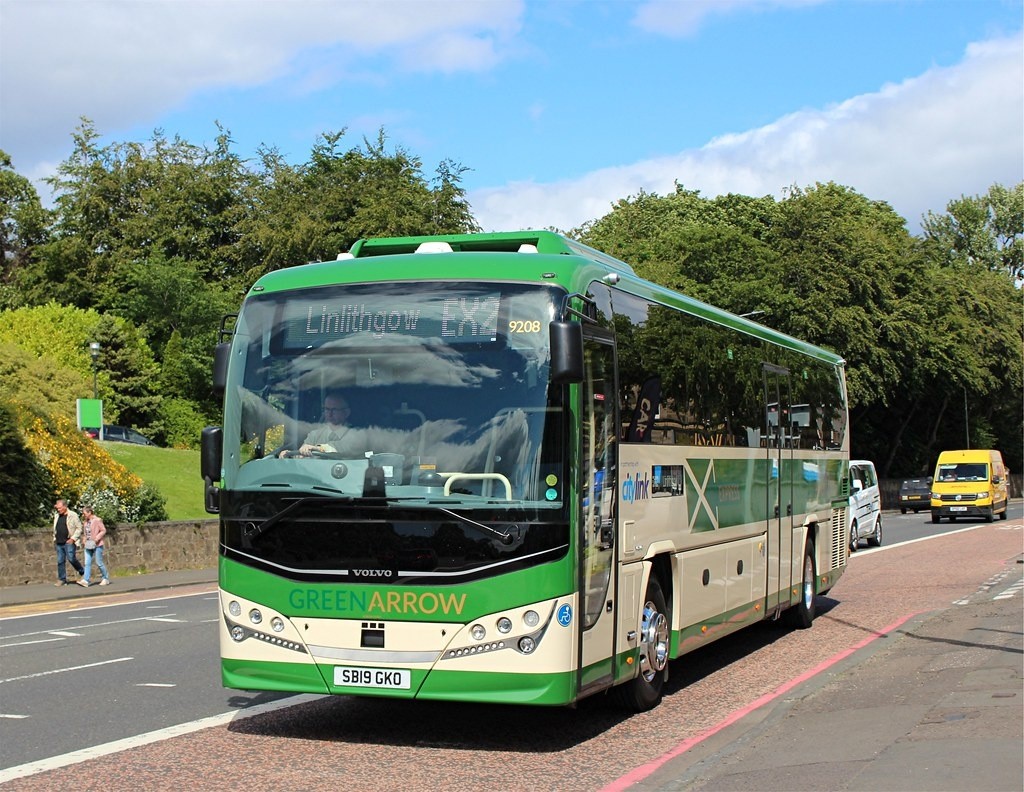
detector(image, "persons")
[76,506,110,588]
[278,390,370,461]
[580,461,605,518]
[948,470,958,479]
[52,499,84,587]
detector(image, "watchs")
[316,444,321,452]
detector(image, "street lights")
[90,343,100,399]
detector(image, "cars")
[898,477,930,514]
[87,423,157,446]
[849,460,883,551]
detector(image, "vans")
[927,450,1009,523]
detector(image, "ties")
[328,433,335,441]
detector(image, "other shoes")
[77,579,88,588]
[76,575,85,584]
[100,578,109,585]
[54,579,67,586]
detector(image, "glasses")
[323,406,347,414]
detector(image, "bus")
[202,232,850,709]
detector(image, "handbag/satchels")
[85,540,95,549]
